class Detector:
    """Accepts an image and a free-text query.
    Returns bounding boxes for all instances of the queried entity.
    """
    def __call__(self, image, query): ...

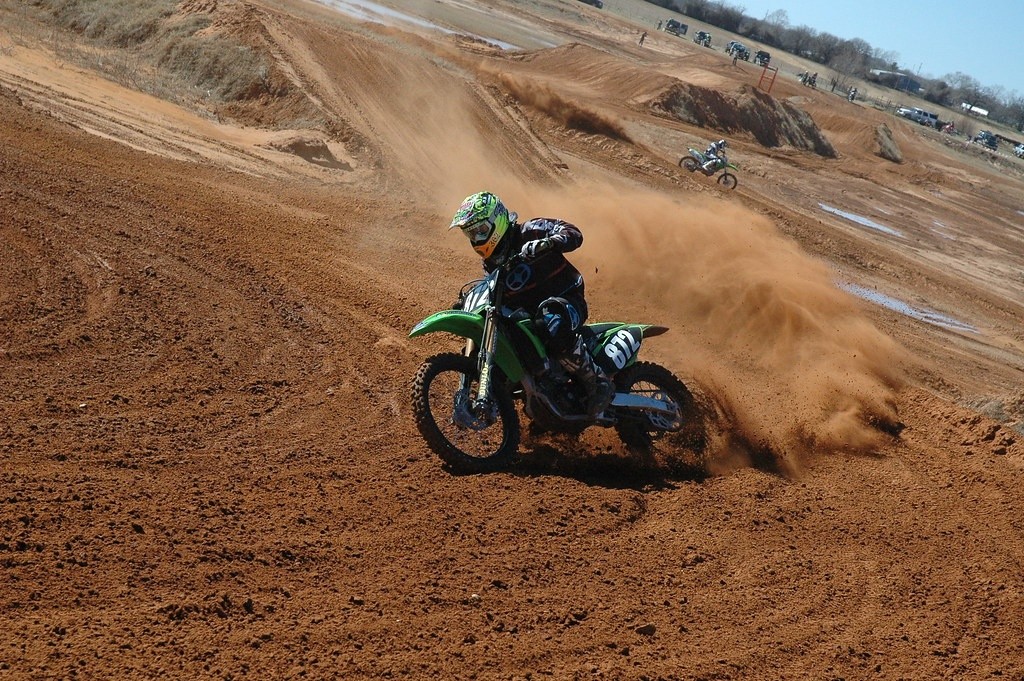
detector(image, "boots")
[702,160,714,171]
[558,334,616,414]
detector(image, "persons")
[801,71,809,83]
[812,72,818,83]
[701,138,728,172]
[656,20,663,30]
[848,86,859,101]
[950,121,955,131]
[638,31,647,47]
[448,191,615,417]
[732,50,740,65]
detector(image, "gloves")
[521,238,553,262]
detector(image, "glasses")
[461,218,493,243]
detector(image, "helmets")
[448,191,518,262]
[719,140,728,149]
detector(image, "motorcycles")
[406,244,706,474]
[850,87,857,101]
[805,77,817,89]
[678,147,739,190]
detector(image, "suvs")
[897,107,938,127]
[974,129,998,151]
[1014,144,1024,159]
[663,18,688,36]
[692,30,712,47]
[753,50,771,66]
[725,39,750,61]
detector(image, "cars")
[577,0,604,9]
